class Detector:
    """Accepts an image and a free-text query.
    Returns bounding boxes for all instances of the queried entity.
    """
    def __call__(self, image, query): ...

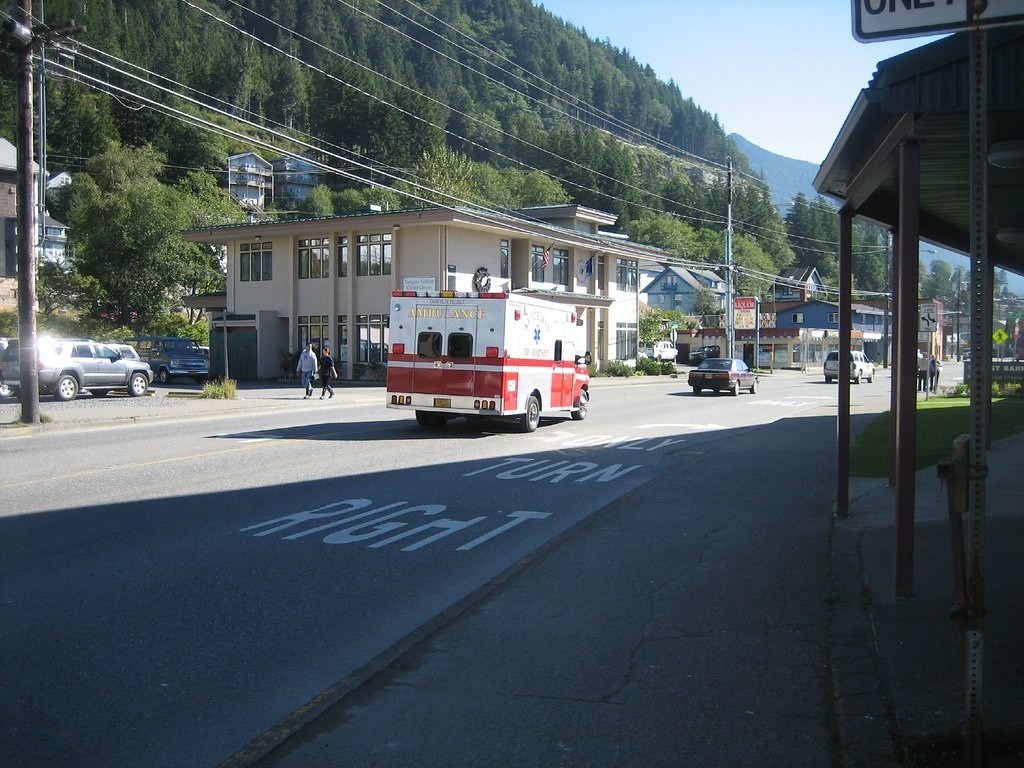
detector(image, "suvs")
[1,334,153,403]
[123,336,213,384]
[689,344,720,364]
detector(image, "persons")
[318,347,338,399]
[918,351,940,392]
[296,343,317,399]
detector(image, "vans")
[823,351,877,385]
[640,340,679,363]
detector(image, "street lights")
[882,248,937,369]
[725,201,796,358]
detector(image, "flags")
[541,247,551,271]
[585,257,593,278]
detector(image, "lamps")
[393,225,401,231]
[255,235,261,241]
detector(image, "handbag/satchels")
[313,373,320,380]
[936,365,944,372]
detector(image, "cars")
[688,358,761,396]
[962,347,971,359]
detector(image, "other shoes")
[320,396,326,400]
[328,393,335,399]
[304,395,309,399]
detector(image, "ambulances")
[382,290,593,433]
